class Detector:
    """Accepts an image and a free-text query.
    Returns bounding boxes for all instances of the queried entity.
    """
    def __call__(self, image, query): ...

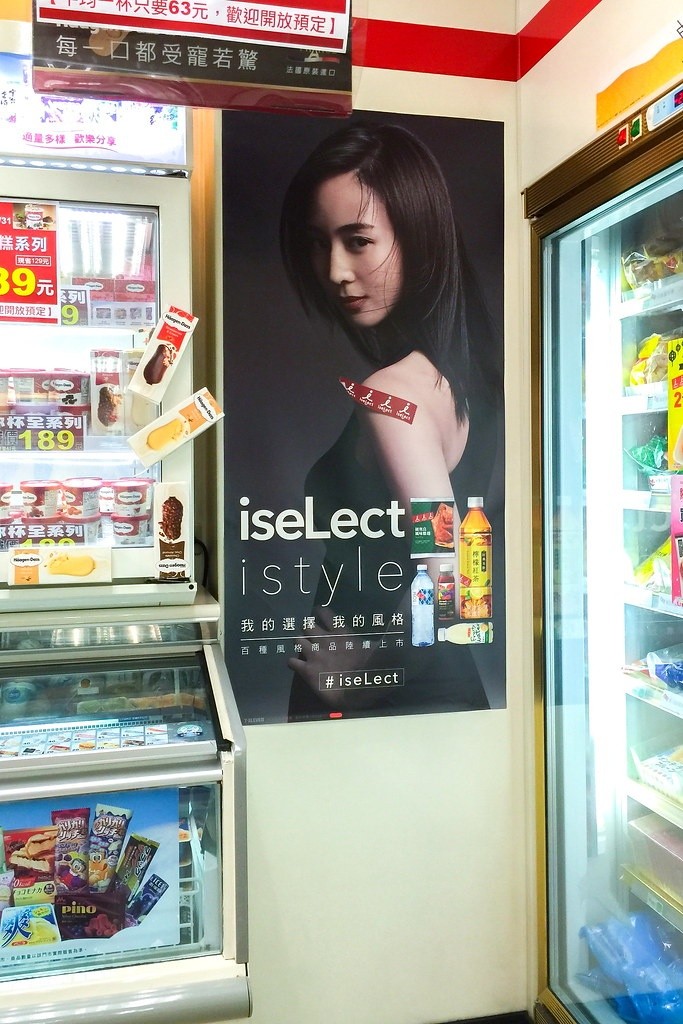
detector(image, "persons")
[277,121,490,723]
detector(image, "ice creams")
[0,348,159,549]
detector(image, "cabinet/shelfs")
[0,151,255,1024]
[520,71,679,1021]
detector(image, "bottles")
[411,563,435,648]
[460,496,492,619]
[438,562,458,622]
[438,623,495,645]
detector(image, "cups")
[0,367,95,436]
[0,473,155,547]
